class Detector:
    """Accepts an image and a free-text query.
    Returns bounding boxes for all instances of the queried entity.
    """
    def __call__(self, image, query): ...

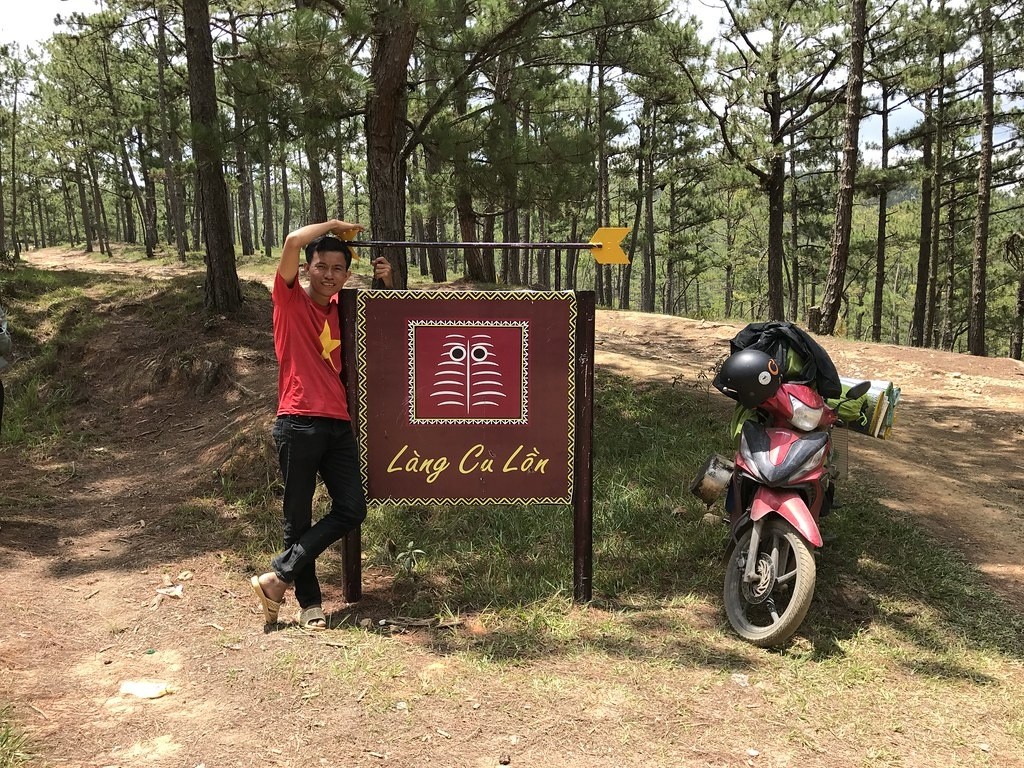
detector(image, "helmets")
[719,349,781,409]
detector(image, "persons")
[251,217,391,633]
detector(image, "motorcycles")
[690,374,872,648]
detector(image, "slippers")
[251,575,282,624]
[298,607,327,631]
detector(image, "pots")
[690,453,734,510]
